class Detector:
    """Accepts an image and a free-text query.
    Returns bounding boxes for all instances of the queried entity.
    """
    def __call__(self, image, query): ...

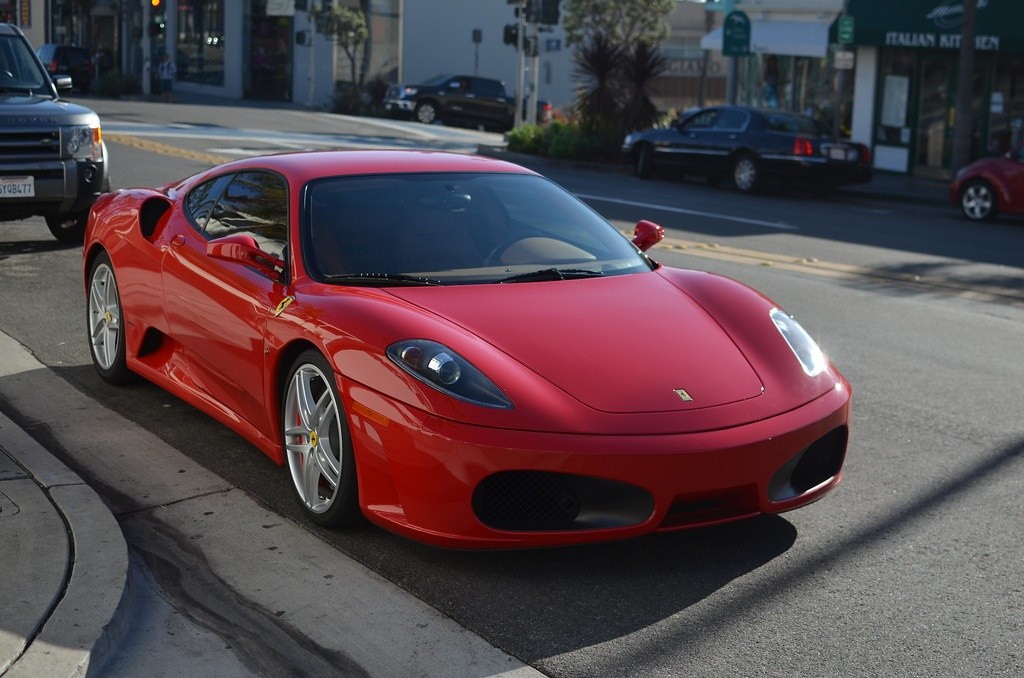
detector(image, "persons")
[459,80,468,91]
[155,53,177,101]
[544,103,580,125]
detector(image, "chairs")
[311,223,356,276]
[398,207,485,272]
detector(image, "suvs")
[0,21,112,246]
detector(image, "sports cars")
[83,148,853,552]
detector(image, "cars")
[380,73,552,132]
[621,107,872,201]
[35,44,95,91]
[951,155,1020,223]
